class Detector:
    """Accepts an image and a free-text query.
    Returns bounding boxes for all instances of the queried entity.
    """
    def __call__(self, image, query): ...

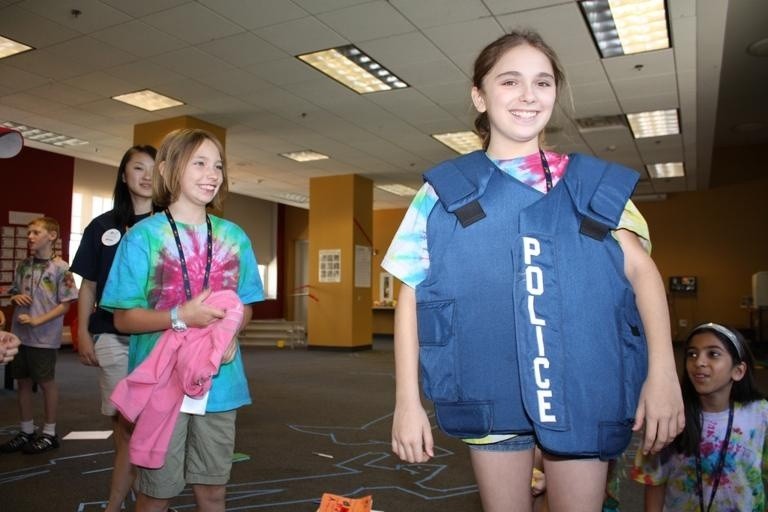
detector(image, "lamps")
[728,119,765,134]
[746,37,767,59]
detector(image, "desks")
[739,304,766,361]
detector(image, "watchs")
[169,305,187,333]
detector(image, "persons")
[532,446,621,511]
[0,219,79,457]
[0,308,7,327]
[67,145,166,511]
[0,331,21,366]
[631,322,767,511]
[380,29,687,512]
[98,125,262,511]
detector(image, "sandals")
[0,430,37,454]
[20,434,60,454]
